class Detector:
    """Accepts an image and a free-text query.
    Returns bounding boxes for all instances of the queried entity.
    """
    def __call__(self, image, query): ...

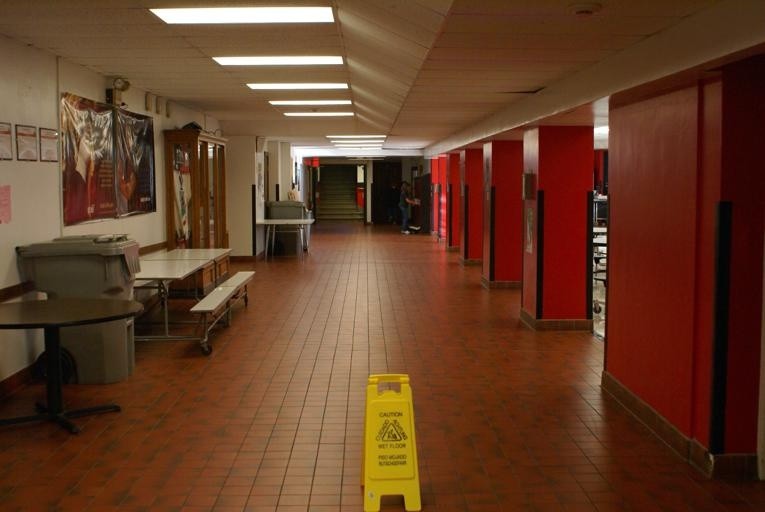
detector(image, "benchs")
[190,268,255,355]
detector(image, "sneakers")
[401,230,410,234]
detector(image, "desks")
[1,300,146,438]
[257,219,316,260]
[136,246,233,354]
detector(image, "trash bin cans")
[266,201,312,255]
[15,234,140,385]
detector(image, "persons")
[387,185,397,224]
[399,181,416,234]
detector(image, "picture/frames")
[0,122,59,163]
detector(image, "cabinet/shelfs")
[169,131,230,298]
